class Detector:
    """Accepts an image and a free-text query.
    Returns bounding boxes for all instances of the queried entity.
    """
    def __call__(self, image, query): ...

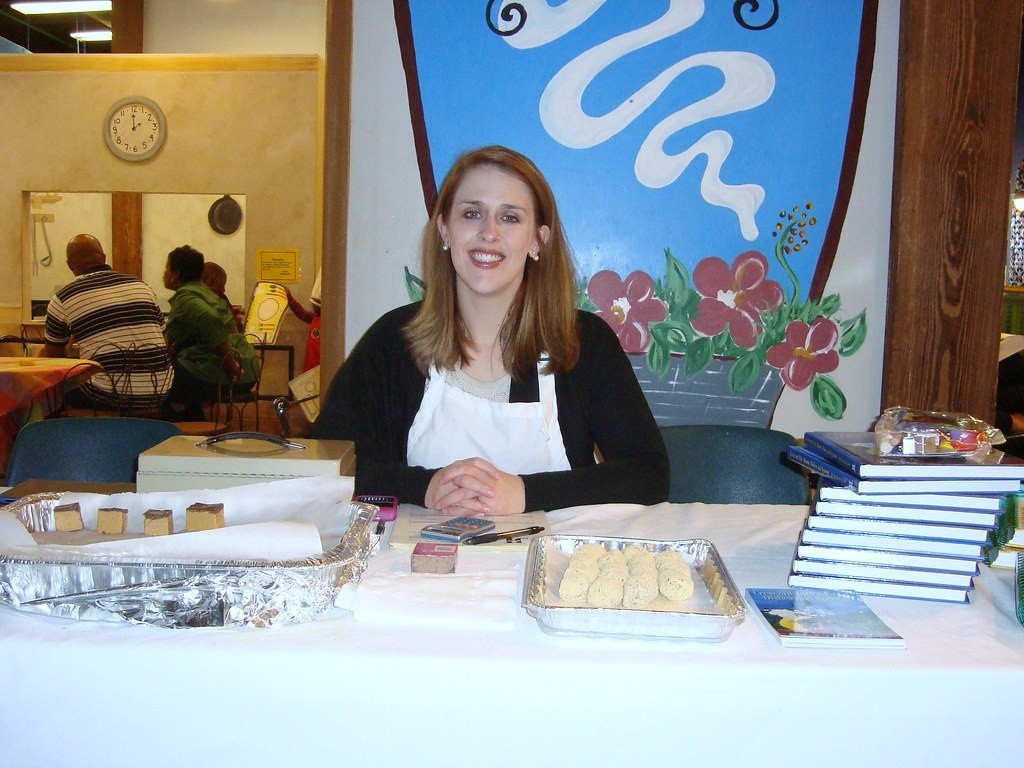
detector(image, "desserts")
[558,543,694,610]
[53,502,84,532]
[142,509,173,536]
[96,508,128,534]
[185,501,227,532]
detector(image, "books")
[787,430,1024,604]
[745,588,905,649]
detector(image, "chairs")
[659,425,813,506]
[20,332,268,436]
[5,417,183,488]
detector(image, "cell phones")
[354,494,399,521]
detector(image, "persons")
[311,145,671,518]
[45,234,175,409]
[285,270,321,371]
[163,245,261,422]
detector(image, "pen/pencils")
[462,525,546,545]
[376,519,386,534]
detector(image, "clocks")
[104,97,166,161]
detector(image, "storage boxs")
[136,431,356,493]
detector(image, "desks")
[0,357,104,416]
[1,502,1024,767]
[0,343,79,359]
